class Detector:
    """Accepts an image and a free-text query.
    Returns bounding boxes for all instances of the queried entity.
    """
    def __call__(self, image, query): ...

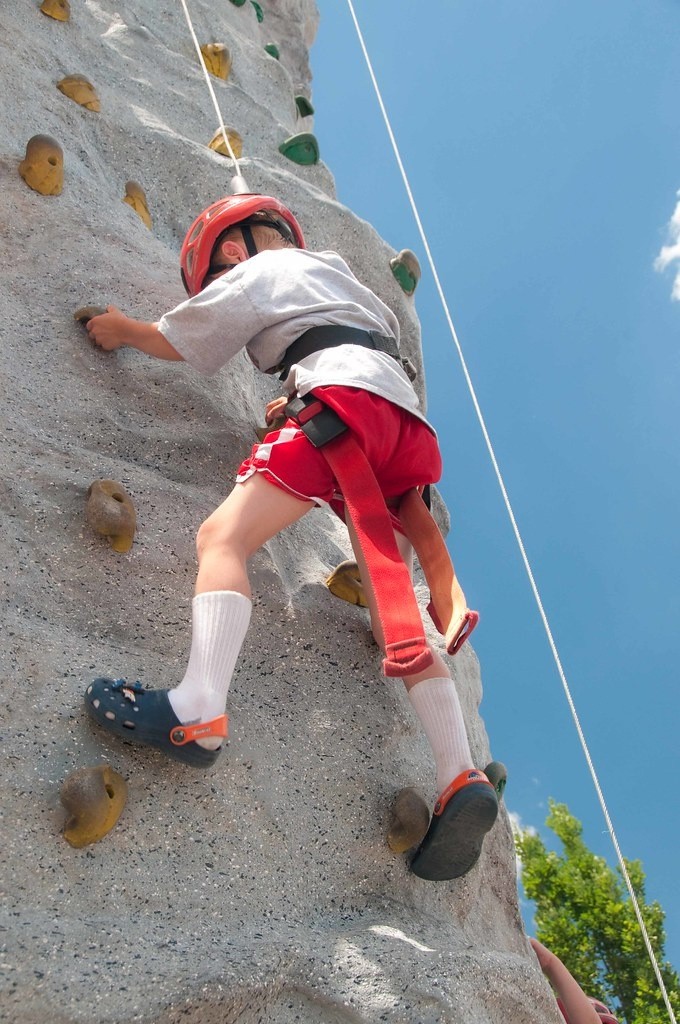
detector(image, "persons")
[85,192,498,880]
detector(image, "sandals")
[83,678,228,766]
[412,768,498,881]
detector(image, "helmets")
[180,192,307,301]
